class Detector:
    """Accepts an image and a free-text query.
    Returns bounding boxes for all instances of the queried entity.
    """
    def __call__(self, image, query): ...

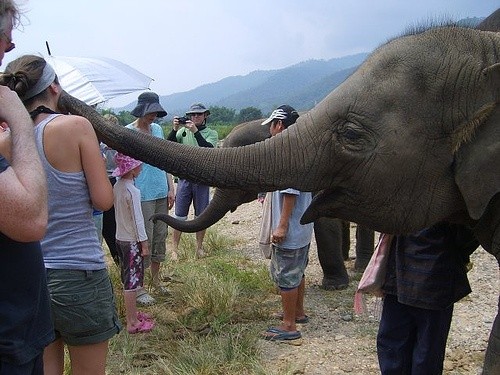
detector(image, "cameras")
[176,117,189,125]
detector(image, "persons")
[99,113,120,267]
[111,150,156,334]
[0,0,57,374]
[0,54,123,375]
[257,104,315,341]
[124,91,176,306]
[167,102,219,264]
[355,231,480,375]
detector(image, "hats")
[130,92,167,118]
[260,104,299,126]
[185,100,211,114]
[111,151,144,177]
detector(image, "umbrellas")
[30,41,156,108]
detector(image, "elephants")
[57,14,500,375]
[148,112,373,291]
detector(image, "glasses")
[3,32,15,52]
[190,113,204,116]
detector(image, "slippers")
[124,311,154,323]
[276,311,309,323]
[127,319,157,334]
[149,279,171,298]
[135,290,157,308]
[259,328,301,340]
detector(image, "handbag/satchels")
[257,196,274,260]
[353,229,394,300]
